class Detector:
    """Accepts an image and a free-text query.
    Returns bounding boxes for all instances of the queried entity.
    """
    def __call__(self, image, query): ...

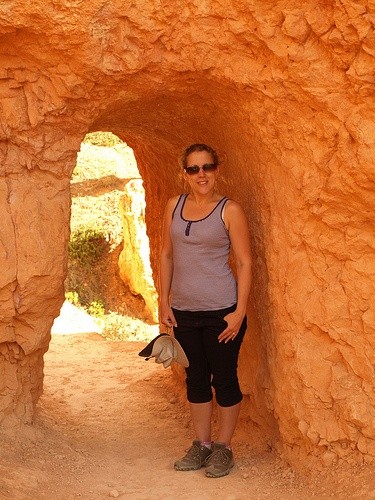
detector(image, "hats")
[138,333,190,368]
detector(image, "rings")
[232,332,235,336]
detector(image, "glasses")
[185,163,216,175]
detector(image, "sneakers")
[174,441,211,471]
[205,442,235,478]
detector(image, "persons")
[159,144,253,478]
